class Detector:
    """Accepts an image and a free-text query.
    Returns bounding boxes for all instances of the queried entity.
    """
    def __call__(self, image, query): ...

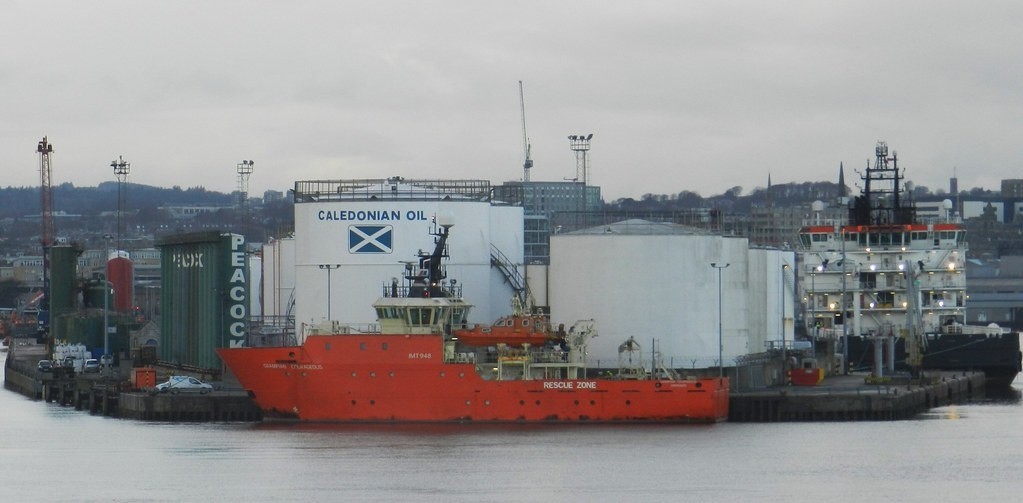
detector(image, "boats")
[793,138,1023,391]
[213,220,732,428]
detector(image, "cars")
[38,360,53,372]
[156,376,214,395]
[84,359,99,372]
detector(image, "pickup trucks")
[100,354,114,366]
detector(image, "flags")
[913,266,922,286]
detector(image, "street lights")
[780,263,792,385]
[319,263,342,320]
[812,265,824,359]
[710,260,730,377]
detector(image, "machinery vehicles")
[236,159,255,217]
[36,134,56,355]
[110,154,133,258]
[517,77,532,179]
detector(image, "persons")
[816,322,821,329]
[607,376,616,380]
[461,318,467,328]
